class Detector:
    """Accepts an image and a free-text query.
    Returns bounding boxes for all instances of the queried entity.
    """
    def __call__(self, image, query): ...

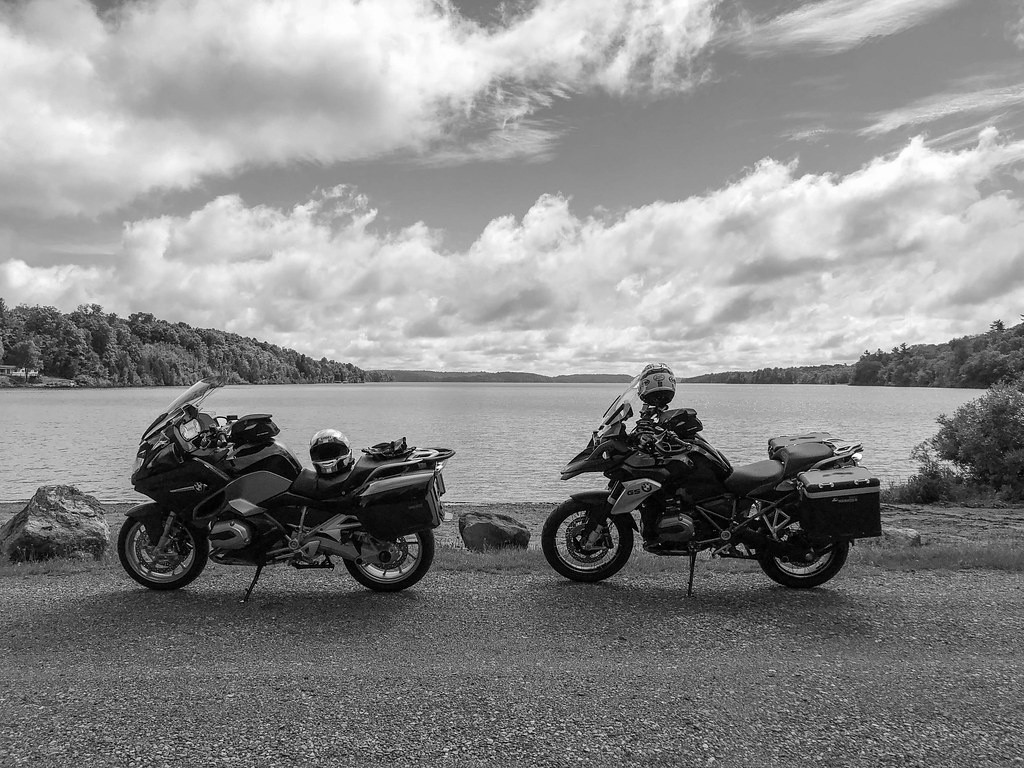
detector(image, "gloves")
[361,437,417,460]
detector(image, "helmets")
[638,363,676,403]
[309,429,355,477]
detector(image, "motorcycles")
[542,372,882,597]
[117,377,456,603]
